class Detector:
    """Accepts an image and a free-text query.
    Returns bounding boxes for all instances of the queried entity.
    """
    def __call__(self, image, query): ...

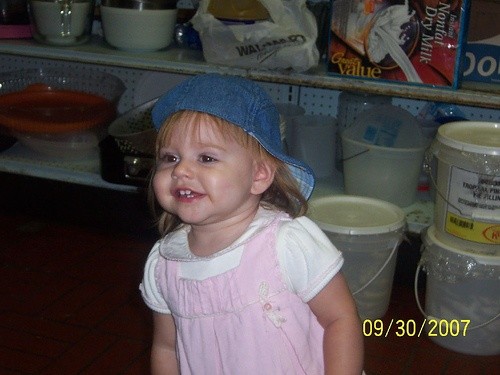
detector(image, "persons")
[139,73,364,375]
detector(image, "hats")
[151,72,316,209]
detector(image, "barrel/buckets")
[414,225,500,357]
[424,121,500,255]
[298,194,407,326]
[336,133,429,208]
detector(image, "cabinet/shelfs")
[0,21,500,284]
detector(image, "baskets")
[107,96,160,157]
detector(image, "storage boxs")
[325,0,473,91]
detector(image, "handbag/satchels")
[191,0,321,73]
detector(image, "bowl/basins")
[0,67,127,159]
[28,1,96,47]
[100,5,179,51]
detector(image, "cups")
[274,104,338,195]
[330,0,419,69]
